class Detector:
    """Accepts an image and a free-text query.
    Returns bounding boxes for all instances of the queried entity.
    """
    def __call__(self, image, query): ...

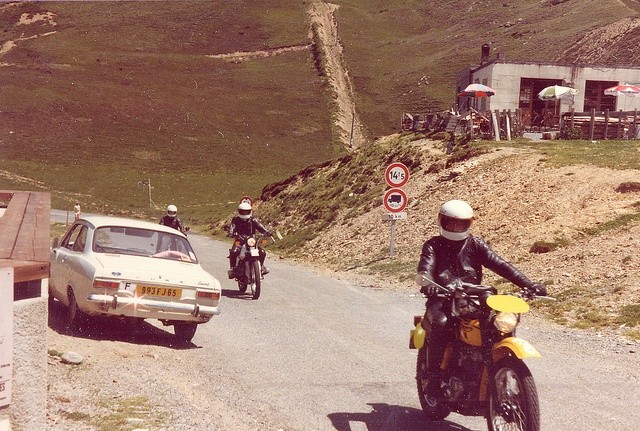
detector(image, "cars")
[49,216,221,342]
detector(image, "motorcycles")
[409,271,557,431]
[226,227,271,298]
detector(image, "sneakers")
[228,269,234,277]
[261,265,270,272]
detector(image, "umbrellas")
[604,83,640,112]
[537,84,576,115]
[457,82,495,110]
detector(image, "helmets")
[437,200,475,242]
[166,205,177,217]
[237,203,253,219]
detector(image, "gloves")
[529,284,547,296]
[424,284,445,299]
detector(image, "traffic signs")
[239,196,253,206]
[383,188,408,213]
[385,162,410,188]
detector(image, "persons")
[416,199,548,400]
[228,202,273,276]
[158,204,188,238]
[73,202,81,221]
